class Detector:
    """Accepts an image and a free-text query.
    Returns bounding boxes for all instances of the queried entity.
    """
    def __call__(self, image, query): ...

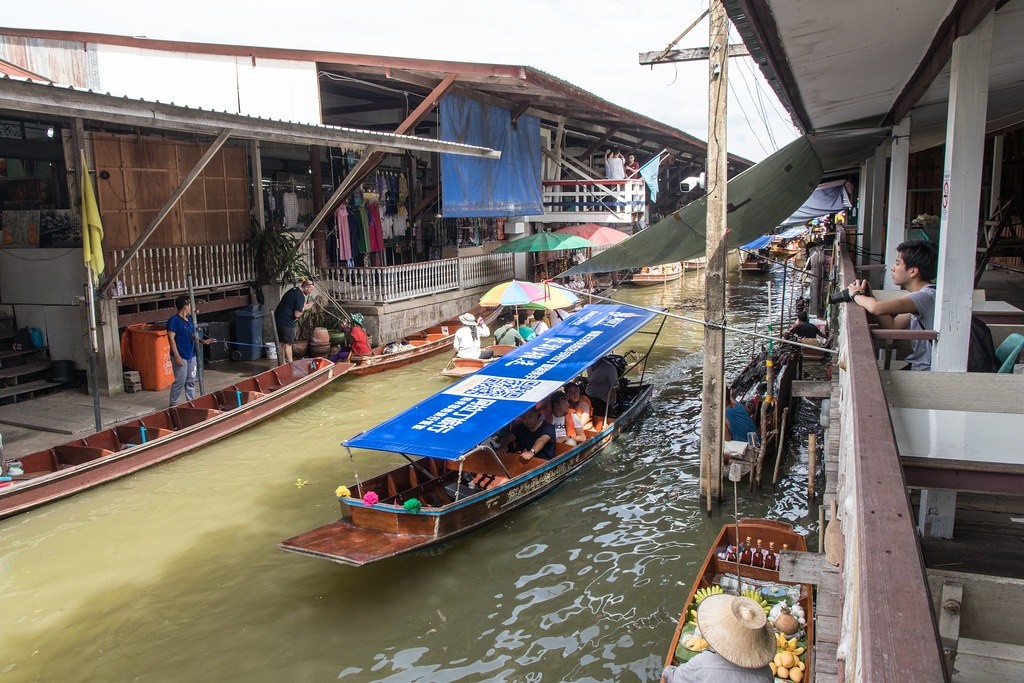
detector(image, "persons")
[604,147,640,210]
[496,356,620,461]
[274,280,315,365]
[662,592,777,683]
[790,312,827,338]
[330,312,373,362]
[325,146,410,260]
[453,313,494,359]
[166,296,218,408]
[493,308,571,346]
[847,239,938,371]
[723,386,758,443]
[827,217,836,232]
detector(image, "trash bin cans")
[120,323,176,391]
[231,309,265,361]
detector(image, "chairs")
[992,332,1024,372]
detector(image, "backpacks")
[915,286,1004,373]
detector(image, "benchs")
[444,481,480,500]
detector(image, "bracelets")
[569,436,572,439]
[851,291,864,301]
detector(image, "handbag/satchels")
[9,324,44,351]
[296,185,314,217]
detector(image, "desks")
[870,300,1024,496]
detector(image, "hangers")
[251,167,410,212]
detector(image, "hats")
[533,310,545,317]
[330,344,342,355]
[696,594,777,670]
[459,313,477,326]
[504,314,515,324]
[348,312,367,333]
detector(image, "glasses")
[307,282,315,285]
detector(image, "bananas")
[688,585,772,625]
[775,632,806,671]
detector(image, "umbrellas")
[520,281,582,311]
[478,279,549,329]
[553,223,630,247]
[489,230,596,280]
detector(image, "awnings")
[544,134,838,356]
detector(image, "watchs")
[530,448,536,455]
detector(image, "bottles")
[752,539,763,568]
[776,544,788,571]
[765,542,776,570]
[740,536,752,565]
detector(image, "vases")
[293,326,345,359]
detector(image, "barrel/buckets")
[265,342,277,360]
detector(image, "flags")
[80,156,105,276]
[639,157,659,203]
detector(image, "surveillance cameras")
[680,177,697,193]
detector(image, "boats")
[792,315,827,361]
[684,257,705,271]
[279,301,669,568]
[520,285,617,321]
[343,306,506,374]
[773,228,807,258]
[660,516,812,683]
[740,236,772,273]
[724,346,798,481]
[630,263,682,286]
[0,358,356,520]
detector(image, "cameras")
[829,281,873,305]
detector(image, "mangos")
[686,634,708,651]
[769,652,804,683]
